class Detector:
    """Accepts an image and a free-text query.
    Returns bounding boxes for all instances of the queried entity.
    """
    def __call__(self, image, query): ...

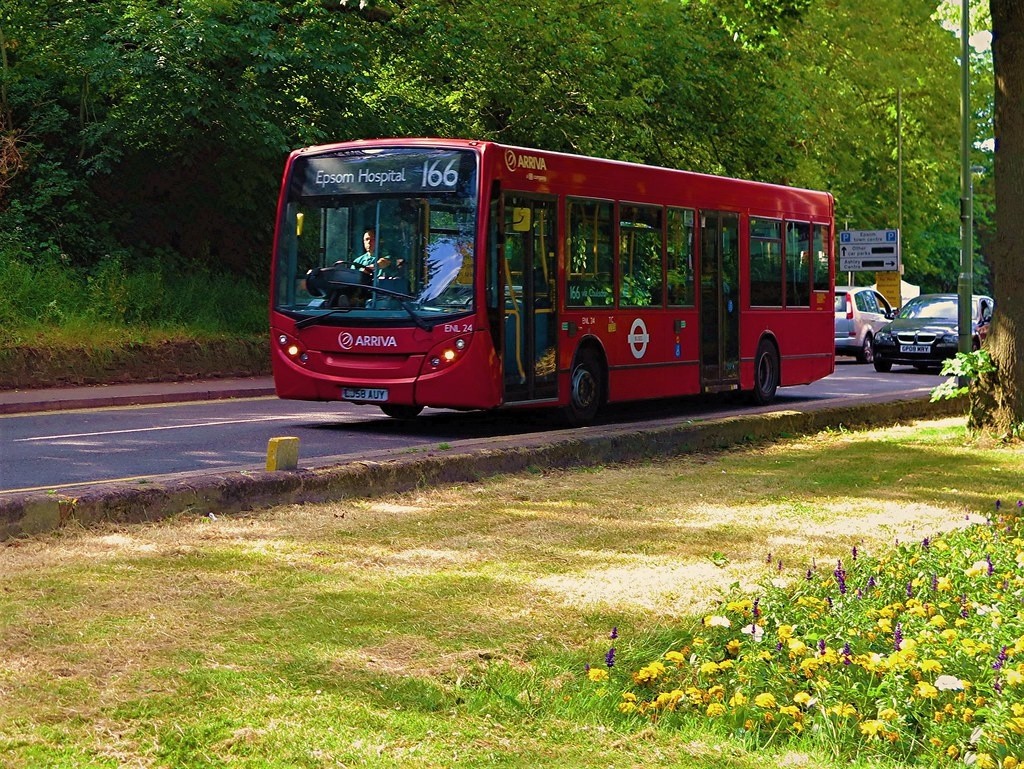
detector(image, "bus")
[269,137,836,425]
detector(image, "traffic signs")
[839,228,899,272]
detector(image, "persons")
[334,226,398,290]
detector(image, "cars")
[834,286,900,365]
[870,294,996,372]
